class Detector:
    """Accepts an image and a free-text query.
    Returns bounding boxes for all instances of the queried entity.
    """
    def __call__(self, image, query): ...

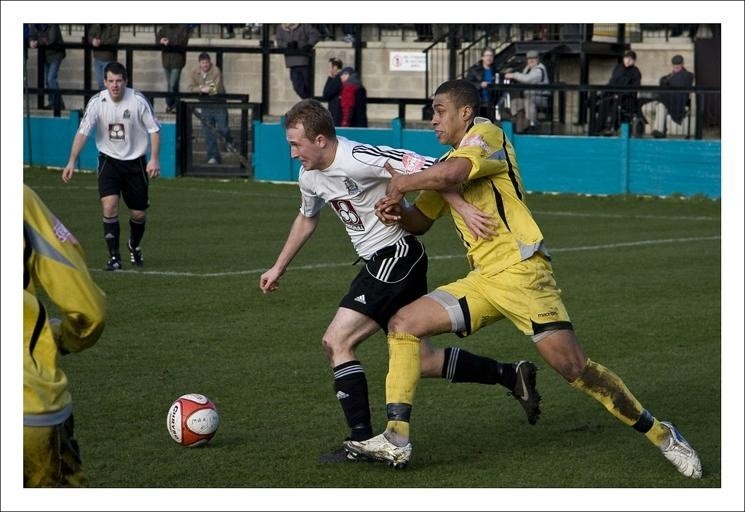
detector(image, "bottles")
[495,73,500,85]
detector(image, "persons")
[275,23,322,99]
[410,23,541,45]
[187,53,237,166]
[341,80,705,482]
[155,24,190,114]
[466,47,553,136]
[323,57,344,127]
[336,66,367,128]
[27,23,67,117]
[85,24,120,90]
[62,62,161,269]
[23,185,111,488]
[259,99,542,462]
[585,49,694,140]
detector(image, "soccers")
[166,393,219,448]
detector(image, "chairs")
[519,89,554,135]
[664,99,691,139]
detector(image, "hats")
[525,48,686,66]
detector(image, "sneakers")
[514,358,545,427]
[104,246,144,271]
[316,438,385,465]
[207,143,237,166]
[654,416,704,481]
[342,435,414,470]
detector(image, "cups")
[207,80,218,97]
[504,79,511,85]
